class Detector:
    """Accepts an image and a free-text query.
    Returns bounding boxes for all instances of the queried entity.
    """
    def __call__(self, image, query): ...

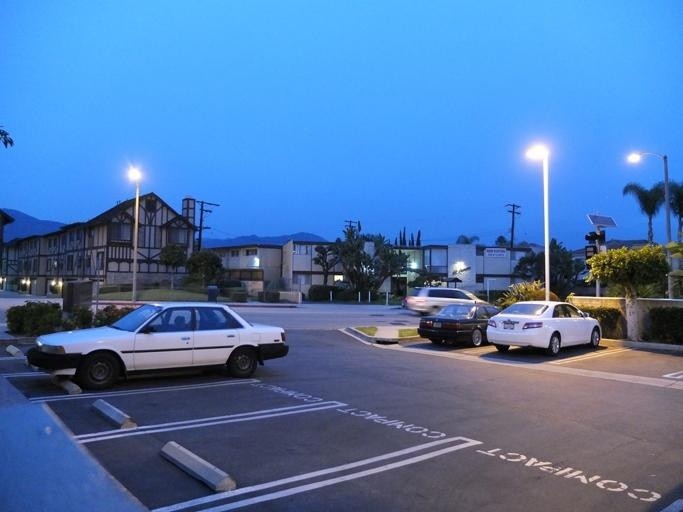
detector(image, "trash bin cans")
[62,281,93,312]
[258,291,280,303]
[665,270,683,299]
[208,286,218,301]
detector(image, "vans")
[404,286,492,315]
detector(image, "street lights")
[526,144,551,300]
[128,168,142,302]
[628,151,672,243]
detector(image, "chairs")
[166,316,233,332]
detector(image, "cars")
[417,304,501,348]
[28,302,291,392]
[486,299,604,356]
